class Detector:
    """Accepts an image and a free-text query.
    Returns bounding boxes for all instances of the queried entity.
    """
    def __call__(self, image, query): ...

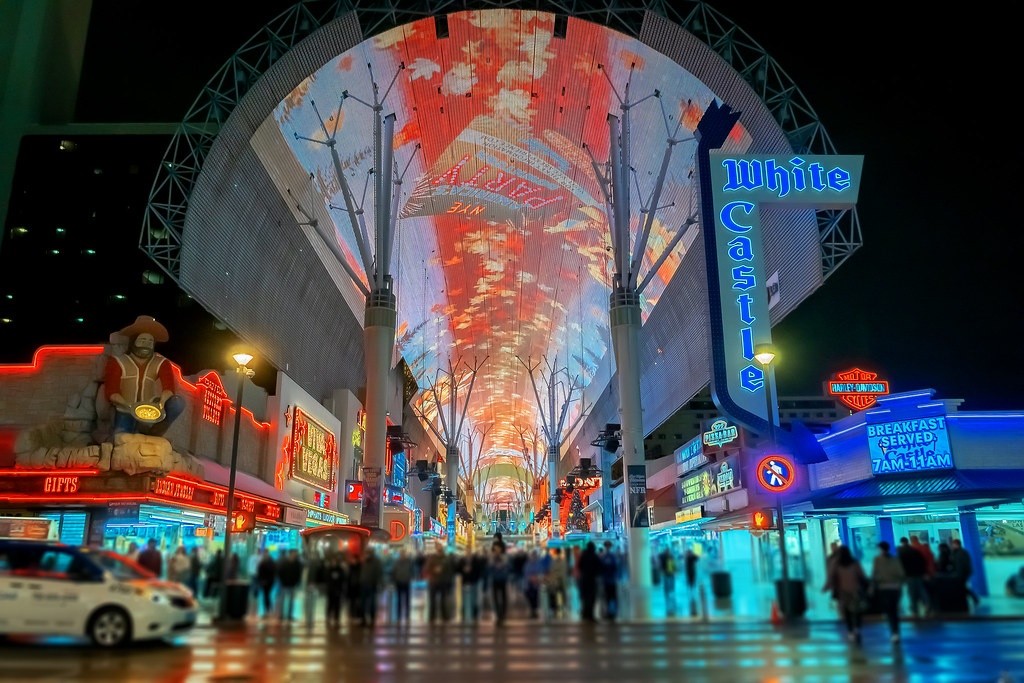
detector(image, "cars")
[0,538,198,649]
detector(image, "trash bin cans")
[711,571,732,600]
[774,578,807,620]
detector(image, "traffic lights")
[234,510,255,532]
[753,509,772,530]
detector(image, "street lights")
[213,353,256,625]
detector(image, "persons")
[120,528,707,637]
[820,539,984,652]
